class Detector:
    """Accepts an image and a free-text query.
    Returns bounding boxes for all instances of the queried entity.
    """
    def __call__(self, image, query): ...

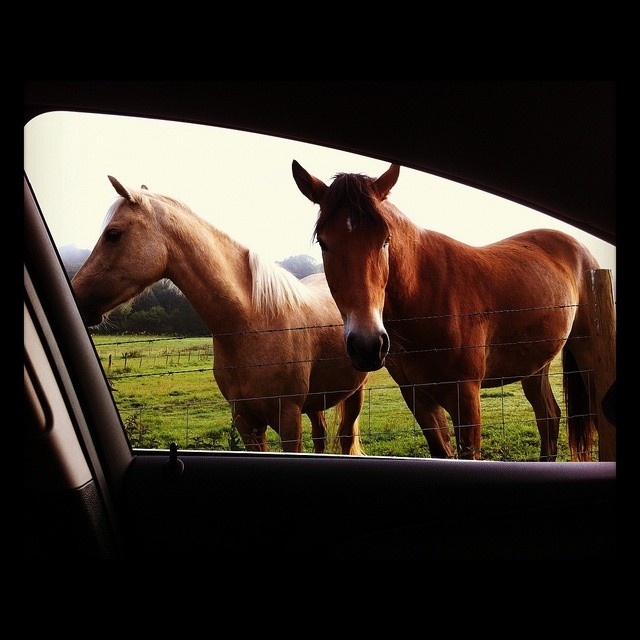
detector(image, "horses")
[291,160,601,460]
[70,174,366,457]
